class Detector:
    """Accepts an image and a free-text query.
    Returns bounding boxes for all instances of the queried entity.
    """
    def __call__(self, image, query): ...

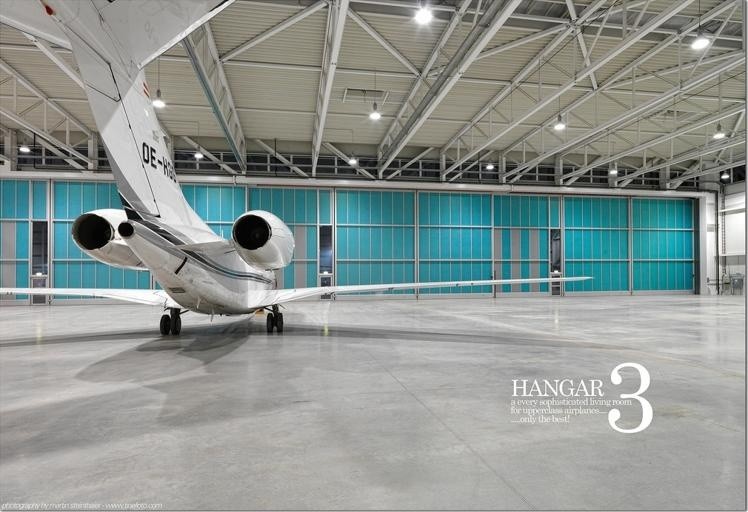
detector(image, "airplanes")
[0,0,594,336]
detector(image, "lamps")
[484,144,494,170]
[151,55,165,108]
[712,72,724,141]
[348,67,380,165]
[721,170,730,180]
[194,120,202,159]
[19,133,30,153]
[553,80,565,131]
[608,141,618,175]
[689,0,709,50]
